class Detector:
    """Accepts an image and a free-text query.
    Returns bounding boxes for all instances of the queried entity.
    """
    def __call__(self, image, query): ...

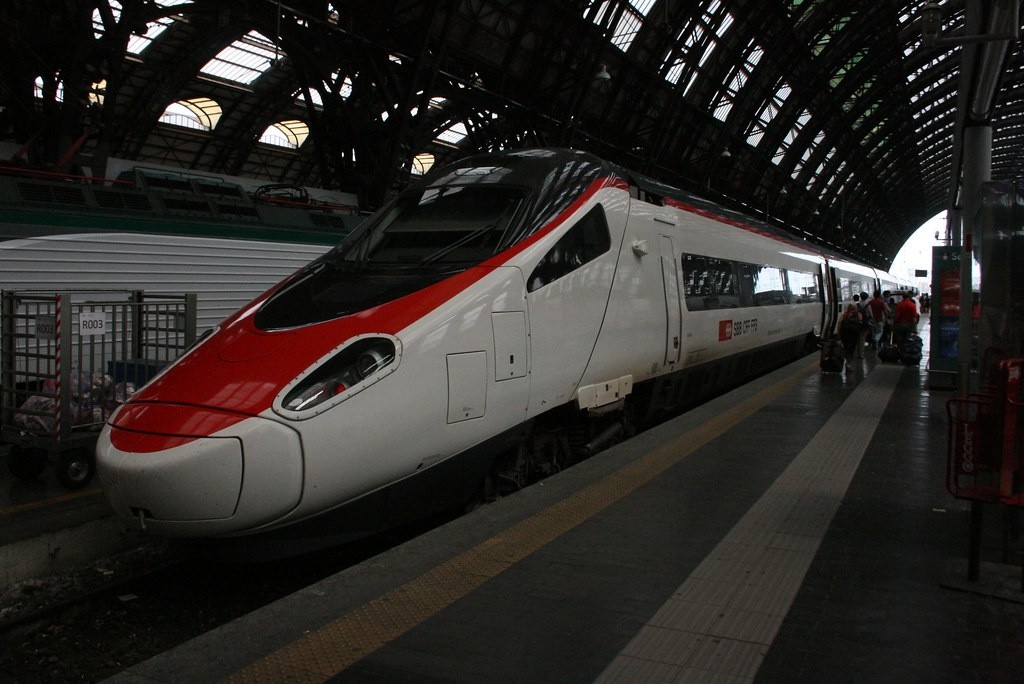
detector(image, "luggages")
[819,333,845,376]
[901,331,923,364]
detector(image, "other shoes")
[845,362,853,372]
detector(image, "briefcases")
[878,330,901,364]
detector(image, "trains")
[93,147,929,543]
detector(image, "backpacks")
[840,316,858,337]
[857,303,873,331]
[870,299,884,321]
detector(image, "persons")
[837,290,930,368]
